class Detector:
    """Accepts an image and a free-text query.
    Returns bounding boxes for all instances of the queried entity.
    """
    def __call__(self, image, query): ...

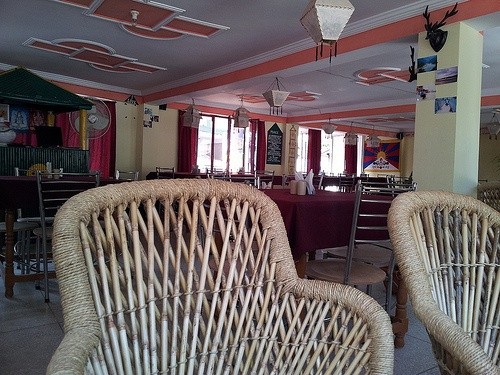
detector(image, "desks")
[0,175,133,300]
[204,189,408,349]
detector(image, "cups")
[297,180,306,195]
[290,180,297,194]
[53,169,61,179]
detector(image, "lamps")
[299,0,356,63]
[487,113,500,136]
[182,76,382,148]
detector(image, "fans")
[69,96,112,139]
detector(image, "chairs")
[0,221,40,275]
[32,171,100,304]
[14,168,66,276]
[387,191,500,375]
[45,178,396,375]
[324,244,393,296]
[477,182,500,213]
[305,180,417,326]
[115,169,140,181]
[146,167,414,196]
